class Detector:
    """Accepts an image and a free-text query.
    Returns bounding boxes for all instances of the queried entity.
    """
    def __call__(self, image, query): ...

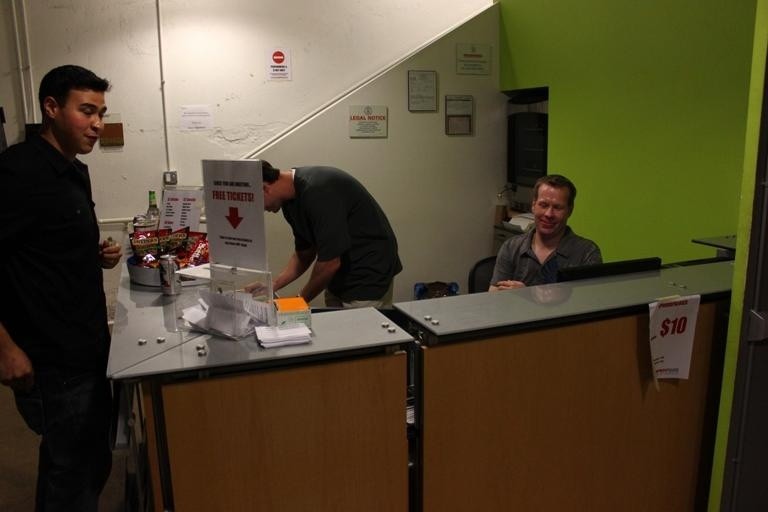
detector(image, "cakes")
[133,220,155,232]
[159,254,182,296]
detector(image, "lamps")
[468,256,498,292]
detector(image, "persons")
[485,171,603,295]
[241,156,404,313]
[0,63,124,512]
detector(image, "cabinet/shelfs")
[146,190,159,217]
